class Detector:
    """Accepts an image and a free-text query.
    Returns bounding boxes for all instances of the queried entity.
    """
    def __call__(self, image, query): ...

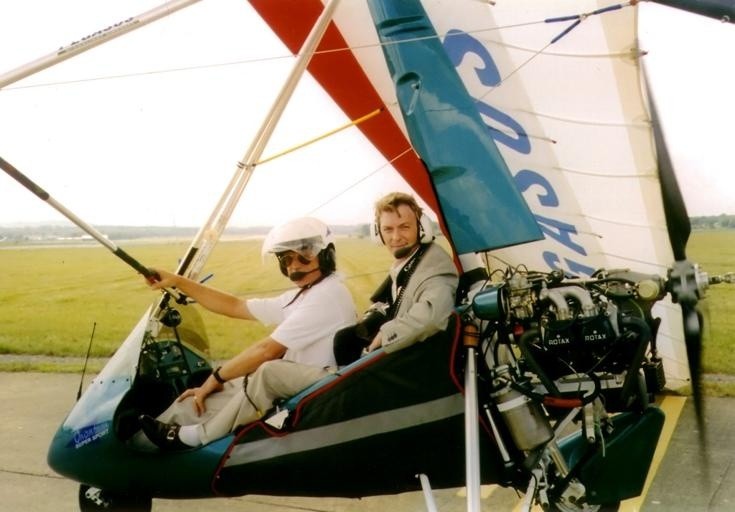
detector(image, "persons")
[138,190,460,451]
[132,216,360,451]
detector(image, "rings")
[192,395,199,401]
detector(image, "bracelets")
[361,346,370,358]
[213,366,227,384]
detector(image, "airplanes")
[0,0,735,512]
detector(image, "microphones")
[290,266,320,281]
[394,241,419,259]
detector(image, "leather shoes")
[138,414,194,450]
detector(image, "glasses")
[280,253,311,268]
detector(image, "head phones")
[278,246,336,278]
[371,191,434,245]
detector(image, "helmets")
[262,218,336,276]
